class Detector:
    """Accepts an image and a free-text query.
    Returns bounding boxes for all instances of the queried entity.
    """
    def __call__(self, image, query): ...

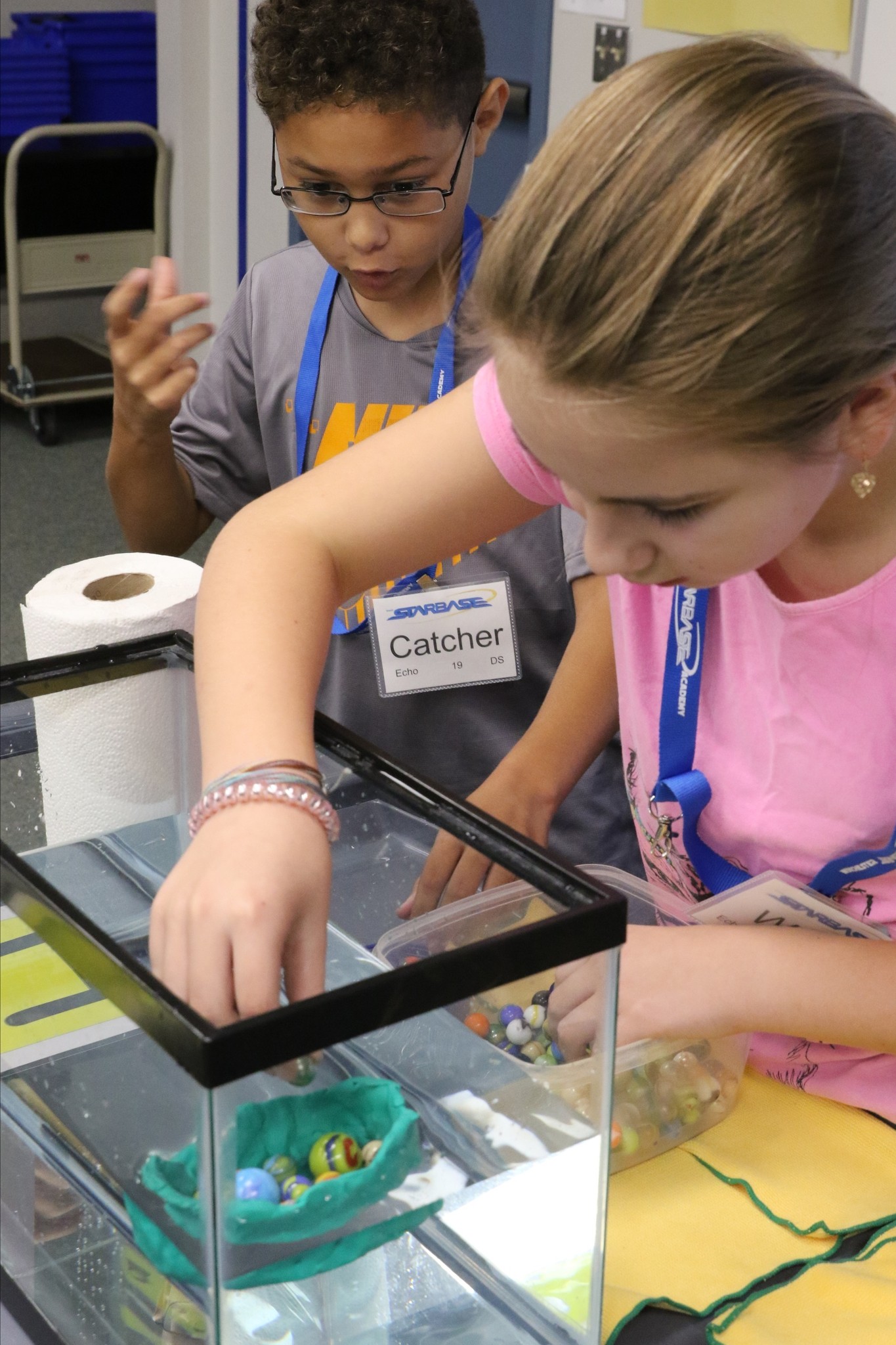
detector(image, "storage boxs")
[3,629,629,1345]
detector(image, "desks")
[434,893,896,1345]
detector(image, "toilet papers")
[18,553,204,847]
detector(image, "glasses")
[271,91,485,217]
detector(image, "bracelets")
[190,760,340,843]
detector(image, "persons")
[104,0,657,954]
[149,38,896,1129]
